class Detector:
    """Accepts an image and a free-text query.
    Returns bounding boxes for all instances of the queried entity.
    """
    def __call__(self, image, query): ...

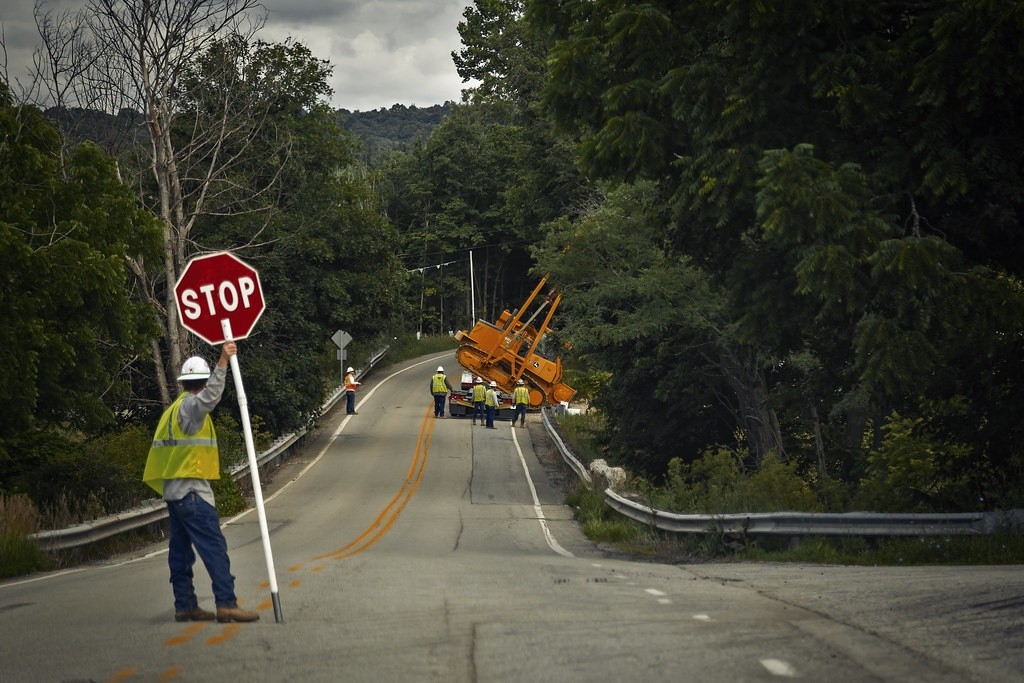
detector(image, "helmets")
[346,367,354,372]
[475,377,483,383]
[437,367,444,371]
[490,381,497,386]
[517,379,524,384]
[176,356,210,380]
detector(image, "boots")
[473,419,476,425]
[511,422,515,427]
[481,418,485,426]
[521,421,526,428]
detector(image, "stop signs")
[174,251,266,346]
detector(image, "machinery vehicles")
[448,235,576,419]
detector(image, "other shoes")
[434,415,438,418]
[486,425,497,430]
[217,605,258,623]
[175,606,215,622]
[346,411,359,415]
[440,415,447,419]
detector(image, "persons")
[430,366,454,419]
[471,377,487,426]
[344,367,362,415]
[143,339,259,623]
[540,332,548,353]
[511,379,530,427]
[485,381,499,429]
